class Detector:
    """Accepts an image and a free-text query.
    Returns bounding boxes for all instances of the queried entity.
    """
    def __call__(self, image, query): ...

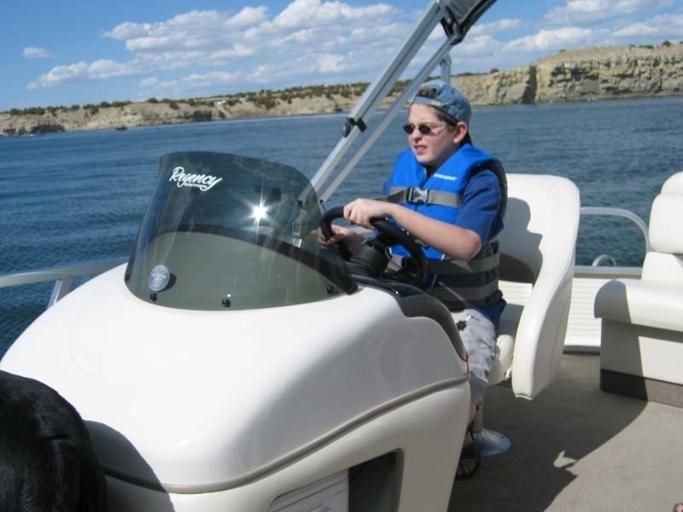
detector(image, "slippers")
[455,428,483,480]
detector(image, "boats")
[0,0,683,512]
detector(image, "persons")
[314,79,507,480]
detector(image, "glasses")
[403,123,447,135]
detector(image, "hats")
[412,79,473,147]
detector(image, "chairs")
[479,171,580,396]
[588,170,683,412]
